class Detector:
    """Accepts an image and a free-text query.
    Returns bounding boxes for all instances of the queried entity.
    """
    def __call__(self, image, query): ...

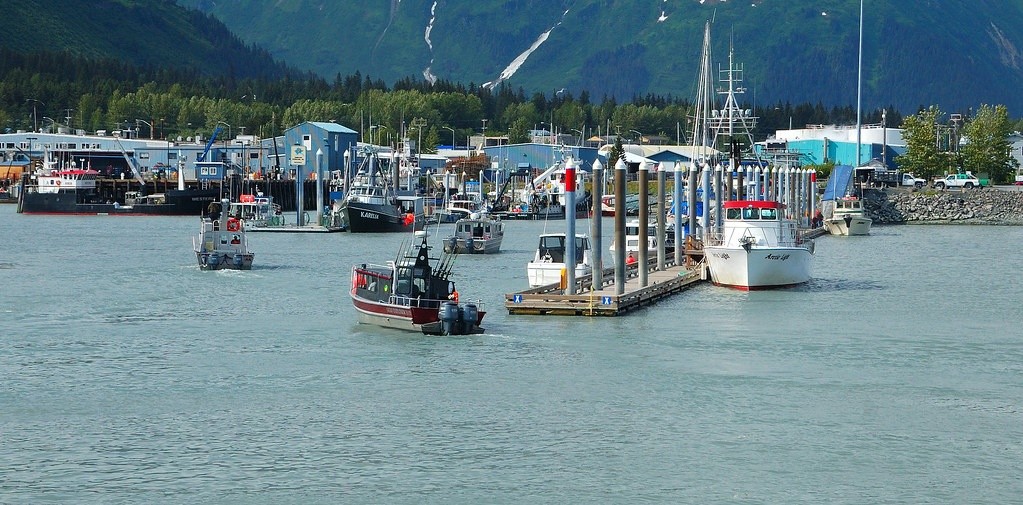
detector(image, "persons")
[627,255,636,269]
[231,236,240,244]
[452,291,458,302]
[208,197,221,231]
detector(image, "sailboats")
[663,18,778,240]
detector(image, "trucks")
[874,170,926,189]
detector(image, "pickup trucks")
[958,174,992,190]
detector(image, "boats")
[821,169,872,236]
[441,195,507,256]
[190,192,255,272]
[608,213,684,267]
[337,109,657,233]
[34,144,99,193]
[701,200,815,292]
[349,191,489,337]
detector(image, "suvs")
[934,171,980,190]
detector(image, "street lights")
[218,121,232,139]
[441,126,456,150]
[41,117,55,133]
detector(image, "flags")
[405,214,414,226]
